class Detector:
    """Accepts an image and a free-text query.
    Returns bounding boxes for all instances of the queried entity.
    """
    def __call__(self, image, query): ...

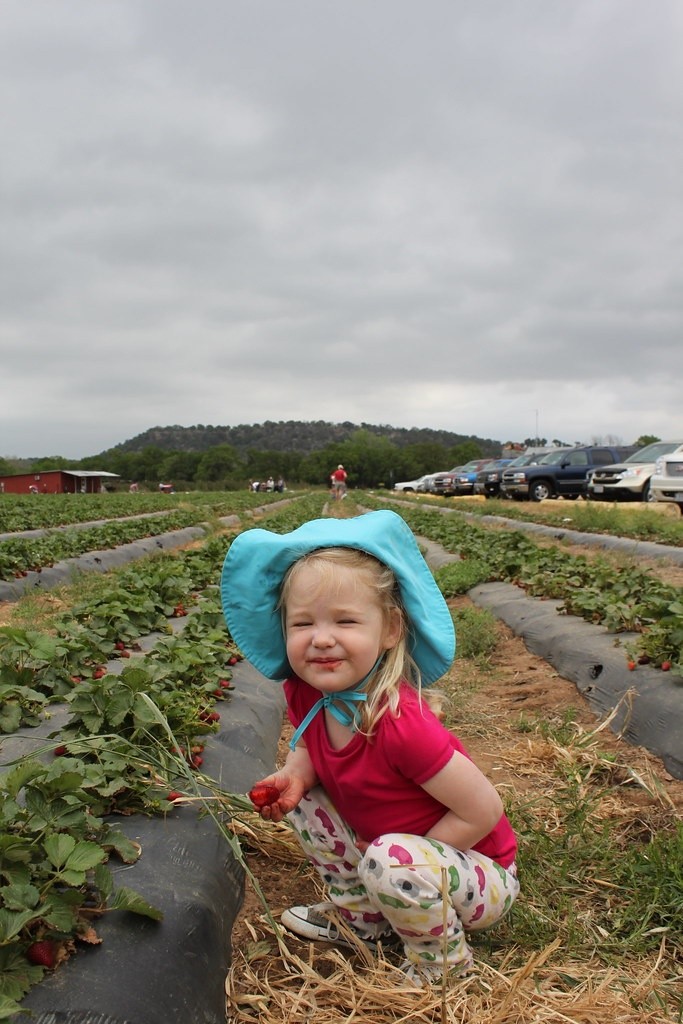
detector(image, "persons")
[130,483,138,493]
[330,464,347,501]
[29,485,38,494]
[221,510,521,989]
[249,475,284,493]
[159,483,164,493]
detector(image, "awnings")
[63,471,120,477]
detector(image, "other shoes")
[280,902,391,956]
[384,952,474,994]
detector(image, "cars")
[394,440,683,502]
[650,444,683,510]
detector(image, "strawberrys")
[27,939,52,964]
[15,553,244,807]
[456,552,671,674]
[250,786,280,809]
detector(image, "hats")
[221,511,455,687]
